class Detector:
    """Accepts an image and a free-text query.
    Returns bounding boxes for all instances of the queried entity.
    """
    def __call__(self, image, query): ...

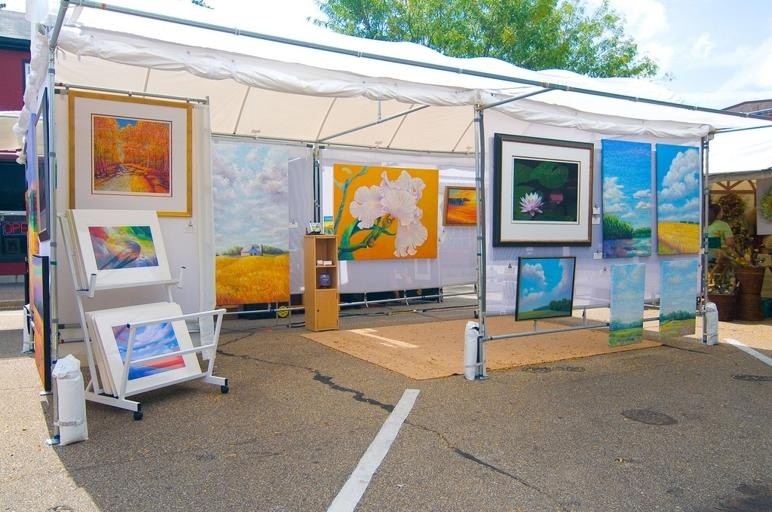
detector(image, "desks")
[215,246,294,322]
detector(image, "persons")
[701,203,735,261]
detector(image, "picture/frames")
[66,210,206,392]
[442,185,478,227]
[515,255,577,321]
[491,132,596,248]
[66,89,193,218]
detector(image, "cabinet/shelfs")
[303,234,340,332]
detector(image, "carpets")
[300,313,663,382]
